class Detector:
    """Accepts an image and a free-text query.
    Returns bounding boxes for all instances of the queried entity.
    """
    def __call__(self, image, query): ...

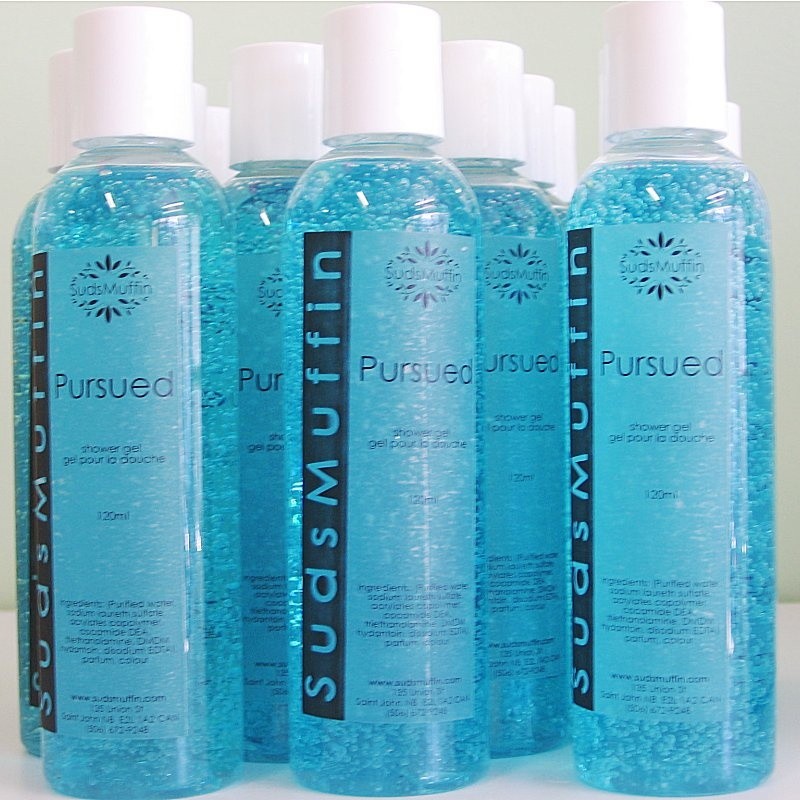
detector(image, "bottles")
[574,3,774,799]
[10,40,747,760]
[29,4,240,800]
[279,3,488,796]
